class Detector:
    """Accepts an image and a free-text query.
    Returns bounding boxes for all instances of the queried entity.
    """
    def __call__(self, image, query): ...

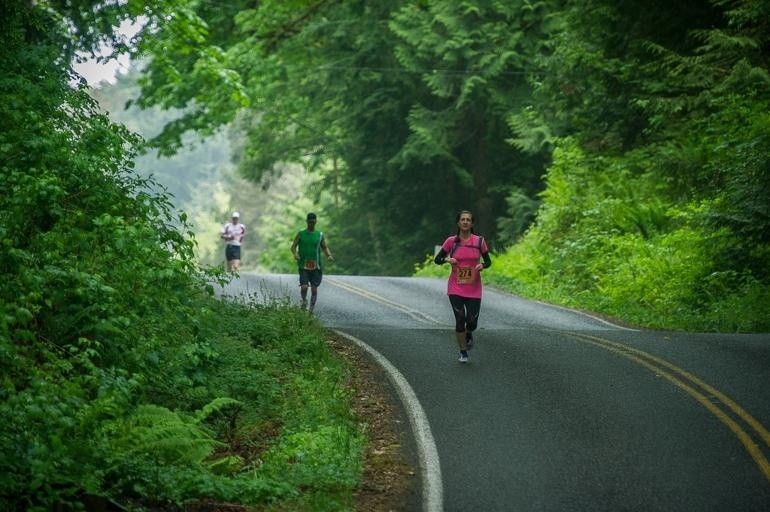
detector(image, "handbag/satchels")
[327,255,331,256]
[228,235,231,240]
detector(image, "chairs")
[458,334,473,363]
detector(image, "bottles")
[231,211,240,218]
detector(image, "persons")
[434,211,492,363]
[221,212,245,279]
[290,213,334,315]
